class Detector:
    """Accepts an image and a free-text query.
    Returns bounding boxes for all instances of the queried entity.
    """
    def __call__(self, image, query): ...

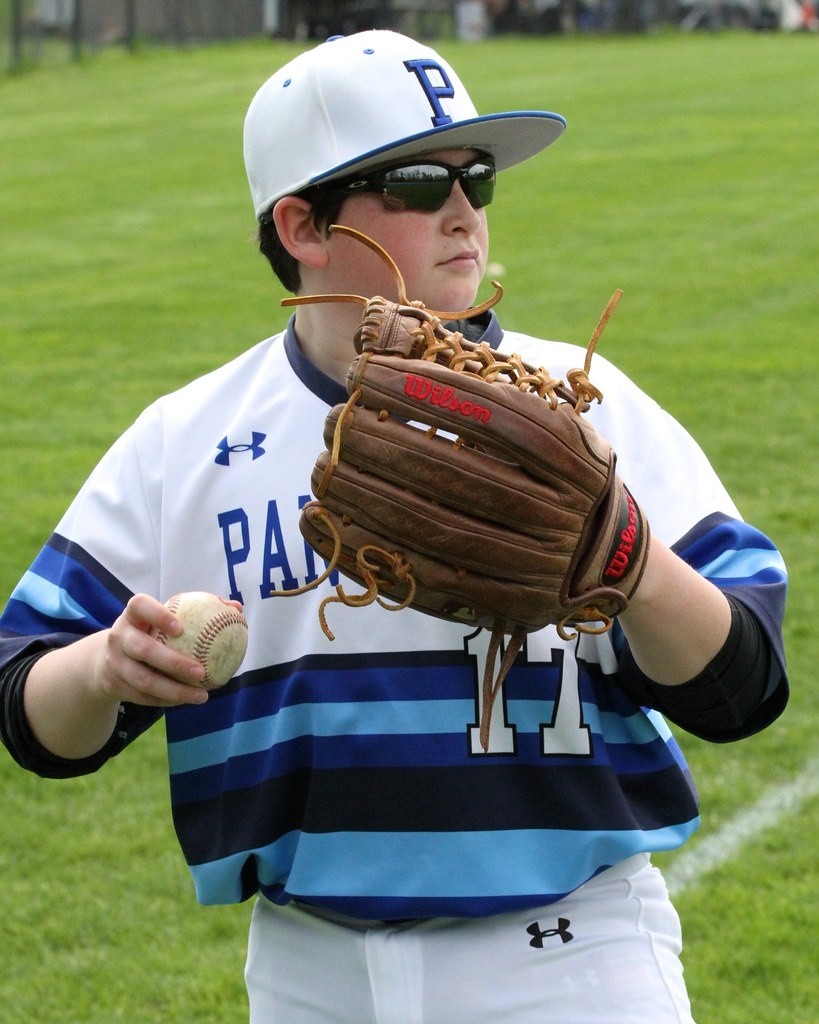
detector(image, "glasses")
[260,156,496,225]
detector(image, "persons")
[0,24,798,1022]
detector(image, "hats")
[241,26,567,222]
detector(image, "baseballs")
[145,593,248,691]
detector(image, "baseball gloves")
[280,224,654,756]
